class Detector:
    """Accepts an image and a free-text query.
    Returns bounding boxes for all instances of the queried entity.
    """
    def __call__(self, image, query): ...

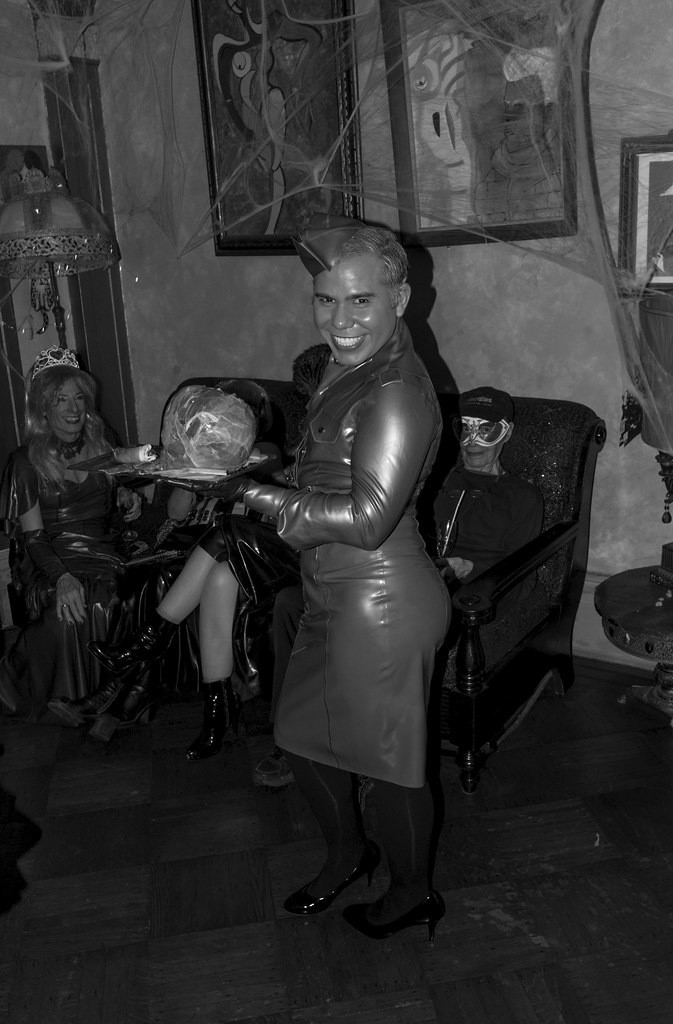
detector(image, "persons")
[251,387,544,786]
[0,344,329,761]
[196,219,453,940]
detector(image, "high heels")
[46,677,131,744]
[342,887,445,942]
[185,675,243,760]
[86,610,180,680]
[108,682,162,729]
[283,838,382,915]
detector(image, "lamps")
[0,167,121,349]
[621,297,673,587]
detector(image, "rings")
[62,605,69,609]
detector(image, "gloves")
[153,474,251,504]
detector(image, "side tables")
[594,565,673,726]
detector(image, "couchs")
[93,377,609,797]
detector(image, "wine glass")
[119,488,138,541]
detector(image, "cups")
[437,519,457,558]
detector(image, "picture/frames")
[377,0,578,247]
[191,0,365,260]
[616,136,673,296]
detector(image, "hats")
[450,385,516,422]
